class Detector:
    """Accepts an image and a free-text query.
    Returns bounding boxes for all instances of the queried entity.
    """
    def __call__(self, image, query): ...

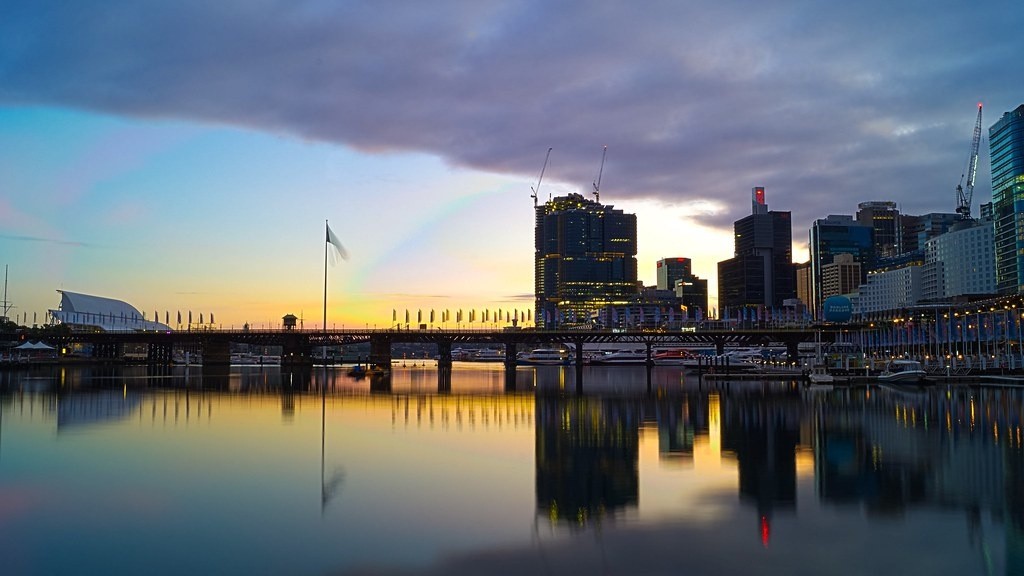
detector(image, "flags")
[327,224,348,264]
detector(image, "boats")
[646,348,696,366]
[504,349,568,365]
[808,367,834,383]
[230,353,281,364]
[682,355,767,374]
[569,350,606,364]
[877,360,928,382]
[592,349,647,363]
[434,347,506,361]
